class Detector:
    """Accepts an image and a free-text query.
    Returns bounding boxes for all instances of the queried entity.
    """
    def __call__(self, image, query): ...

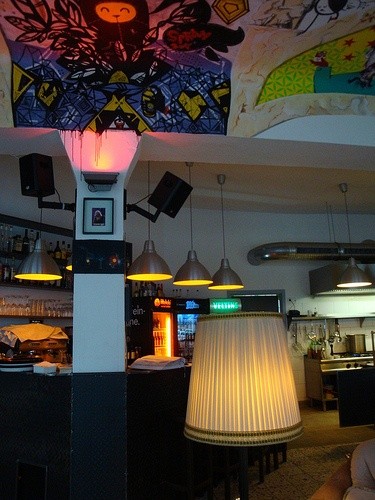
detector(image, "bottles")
[46,299,72,317]
[17,279,36,287]
[177,328,195,339]
[134,281,144,297]
[0,224,8,254]
[153,318,164,356]
[11,256,17,284]
[8,225,22,254]
[172,288,200,298]
[29,230,40,253]
[62,264,68,288]
[47,242,55,260]
[177,318,196,329]
[36,280,61,289]
[157,284,164,297]
[178,340,194,361]
[0,255,10,284]
[61,241,72,260]
[54,240,61,260]
[144,281,157,297]
[22,228,29,254]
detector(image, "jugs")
[310,342,321,359]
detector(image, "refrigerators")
[126,297,210,363]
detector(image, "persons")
[92,208,105,226]
[342,439,375,500]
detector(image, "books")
[33,361,73,373]
[128,355,185,370]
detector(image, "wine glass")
[5,295,31,315]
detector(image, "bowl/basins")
[328,355,340,360]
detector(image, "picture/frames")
[82,197,114,235]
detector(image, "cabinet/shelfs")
[0,213,133,319]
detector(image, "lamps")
[172,162,214,287]
[336,184,372,288]
[14,209,63,281]
[208,174,245,290]
[127,161,173,281]
[183,311,305,500]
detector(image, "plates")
[0,359,43,373]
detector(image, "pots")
[346,334,366,353]
